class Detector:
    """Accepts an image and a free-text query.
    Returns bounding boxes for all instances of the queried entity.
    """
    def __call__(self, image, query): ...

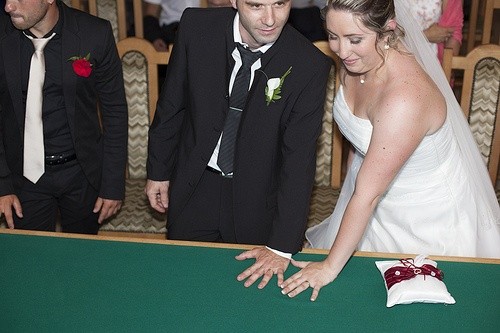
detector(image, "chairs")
[442,44,500,201]
[303,40,355,249]
[72,0,144,43]
[465,0,500,55]
[97,37,173,237]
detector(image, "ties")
[217,42,264,176]
[22,31,56,185]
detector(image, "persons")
[0,0,128,237]
[142,0,330,50]
[280,0,500,302]
[144,0,332,290]
[391,0,464,90]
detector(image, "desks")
[0,228,500,333]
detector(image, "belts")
[44,153,77,168]
[206,165,234,177]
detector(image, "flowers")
[258,67,293,106]
[65,53,94,77]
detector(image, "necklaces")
[359,78,374,85]
